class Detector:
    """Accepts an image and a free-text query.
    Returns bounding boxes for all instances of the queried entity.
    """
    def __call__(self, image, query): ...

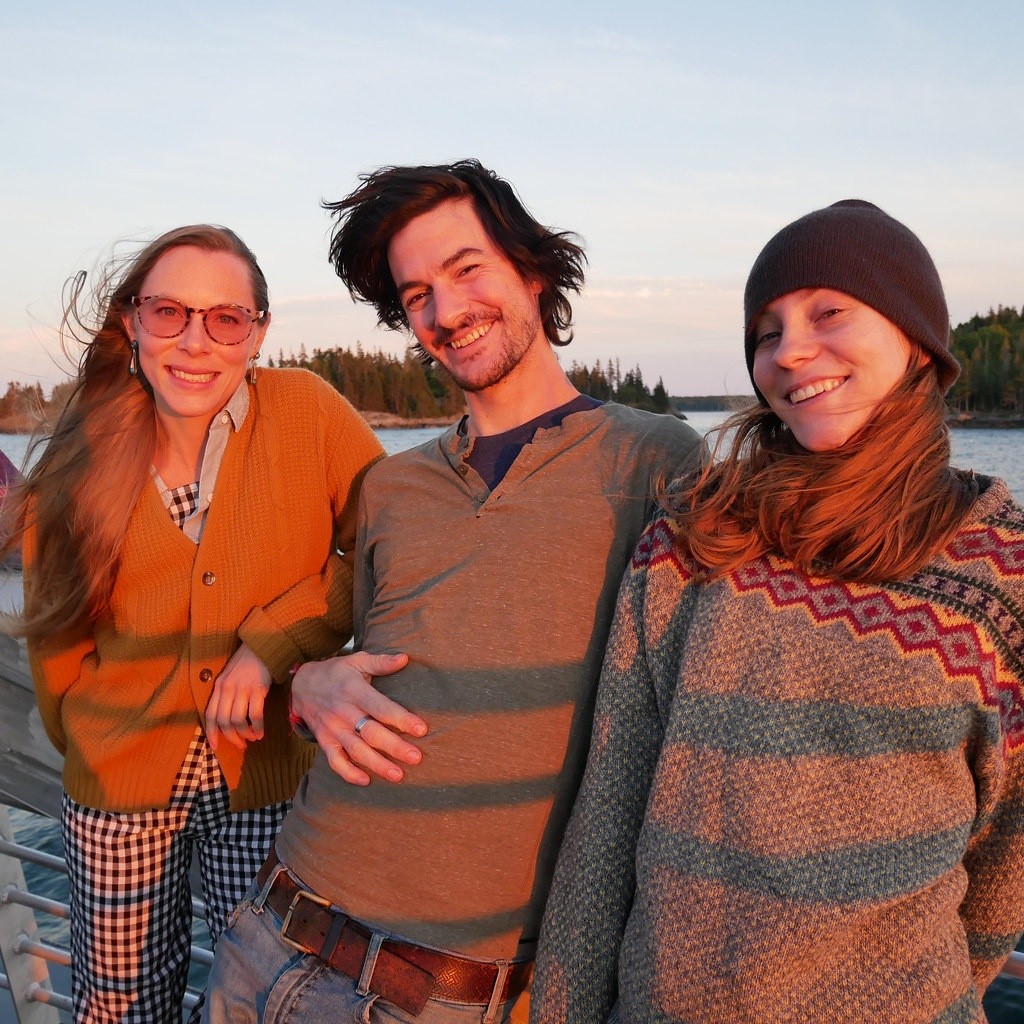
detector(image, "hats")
[742,198,962,423]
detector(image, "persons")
[200,158,712,1024]
[528,200,1024,1024]
[22,223,389,1024]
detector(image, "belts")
[254,843,535,1018]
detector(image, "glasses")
[131,296,264,346]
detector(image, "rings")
[354,715,375,736]
